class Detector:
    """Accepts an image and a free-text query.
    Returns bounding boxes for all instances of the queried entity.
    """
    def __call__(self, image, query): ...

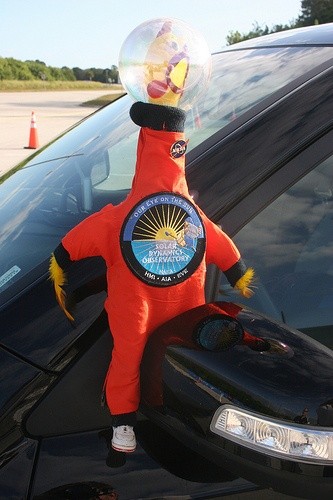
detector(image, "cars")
[1,21,333,500]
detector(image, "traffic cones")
[27,110,40,150]
